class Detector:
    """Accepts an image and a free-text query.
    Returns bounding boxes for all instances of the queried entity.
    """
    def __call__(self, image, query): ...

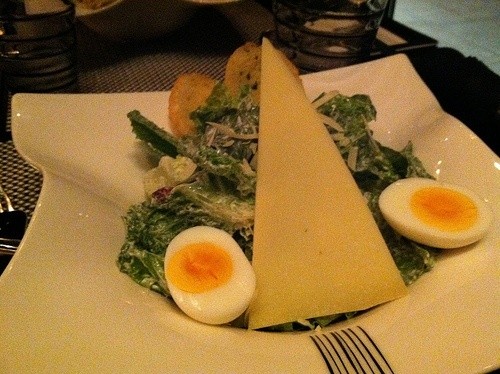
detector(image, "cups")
[0,0,77,93]
[272,0,388,74]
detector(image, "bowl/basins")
[73,0,239,40]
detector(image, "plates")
[0,53,500,374]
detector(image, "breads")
[245,37,407,333]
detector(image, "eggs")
[378,177,491,249]
[163,226,254,325]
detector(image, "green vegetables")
[112,81,439,332]
